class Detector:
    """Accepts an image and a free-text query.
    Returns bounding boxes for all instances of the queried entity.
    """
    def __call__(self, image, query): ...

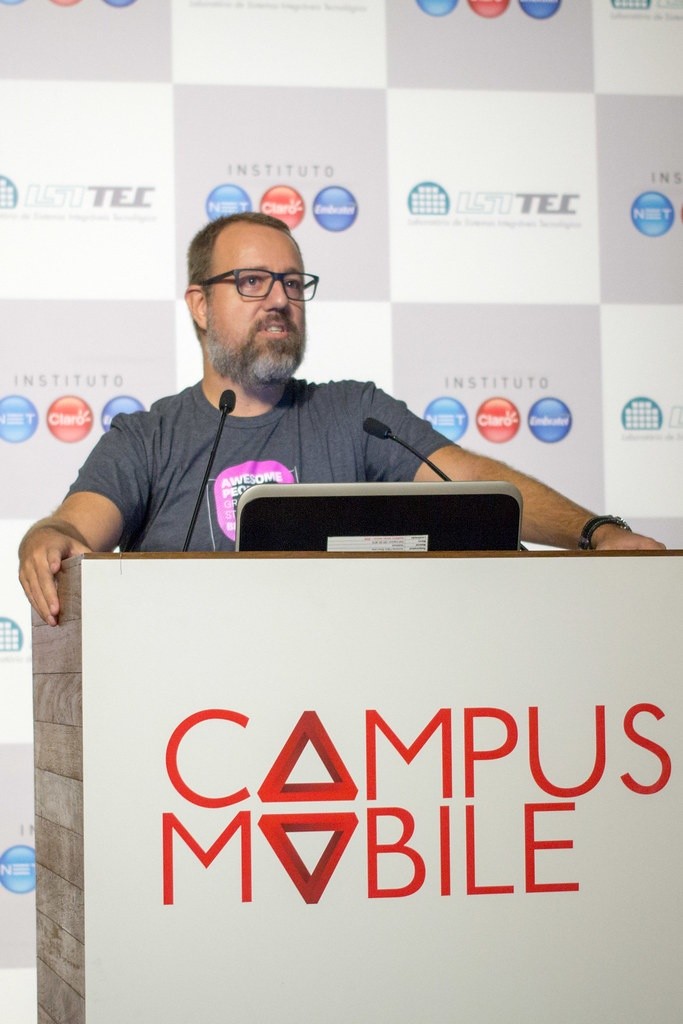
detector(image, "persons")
[19,212,668,626]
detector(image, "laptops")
[237,481,522,552]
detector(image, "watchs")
[579,515,632,550]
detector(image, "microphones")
[182,390,236,551]
[363,417,454,484]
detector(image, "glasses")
[200,267,320,301]
